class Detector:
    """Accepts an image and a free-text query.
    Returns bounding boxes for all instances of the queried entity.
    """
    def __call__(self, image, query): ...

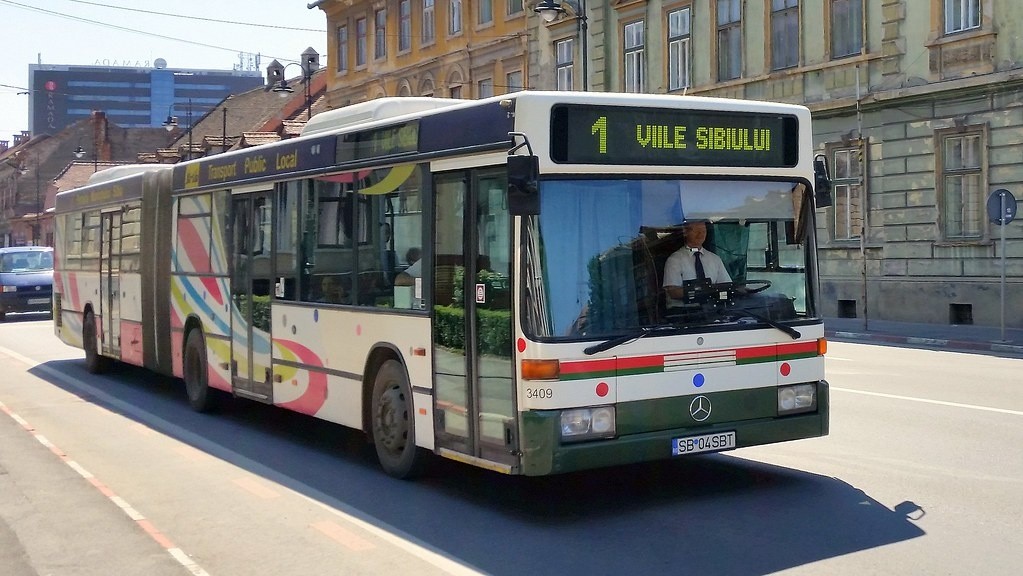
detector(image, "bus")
[54,88,834,483]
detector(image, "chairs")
[16,259,28,268]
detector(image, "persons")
[394,258,422,286]
[316,275,348,305]
[663,220,733,310]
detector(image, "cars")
[0,246,54,318]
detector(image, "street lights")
[532,0,589,91]
[72,133,99,173]
[270,58,313,120]
[160,97,193,161]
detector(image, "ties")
[694,252,705,279]
